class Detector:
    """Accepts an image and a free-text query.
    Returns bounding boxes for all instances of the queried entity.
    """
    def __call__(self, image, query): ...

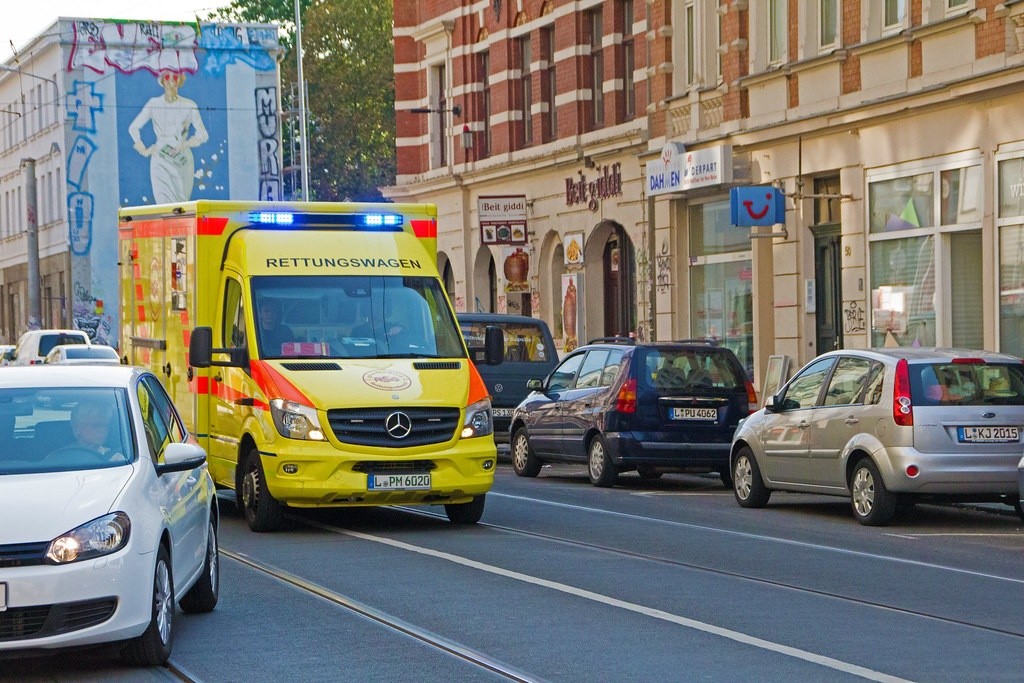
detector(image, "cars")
[0,328,222,664]
[726,346,1024,524]
[505,337,760,487]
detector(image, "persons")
[45,397,126,461]
[350,292,407,342]
[129,70,209,204]
[243,297,295,350]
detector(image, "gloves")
[133,141,159,157]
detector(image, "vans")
[454,309,560,446]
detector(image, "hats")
[187,136,200,148]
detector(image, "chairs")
[350,293,399,339]
[655,366,713,388]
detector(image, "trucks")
[113,199,500,533]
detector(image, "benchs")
[0,414,73,458]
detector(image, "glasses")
[164,73,179,82]
[262,309,276,314]
